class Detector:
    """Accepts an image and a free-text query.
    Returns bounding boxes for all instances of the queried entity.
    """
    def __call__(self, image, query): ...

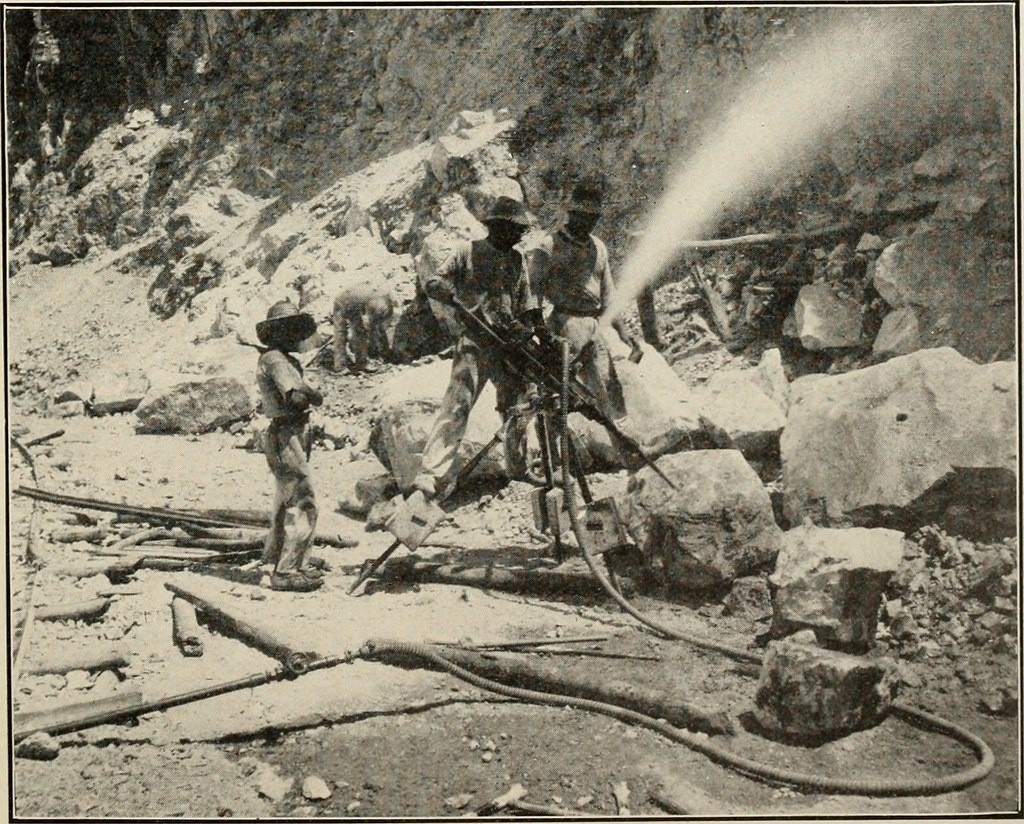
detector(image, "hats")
[559,186,605,214]
[479,196,530,228]
[255,301,317,344]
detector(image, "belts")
[554,306,597,316]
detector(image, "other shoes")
[406,478,436,500]
[506,468,546,487]
[551,466,574,486]
[269,570,324,592]
[623,439,667,473]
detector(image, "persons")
[528,180,668,469]
[249,299,325,592]
[408,195,547,497]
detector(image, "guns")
[451,294,681,493]
